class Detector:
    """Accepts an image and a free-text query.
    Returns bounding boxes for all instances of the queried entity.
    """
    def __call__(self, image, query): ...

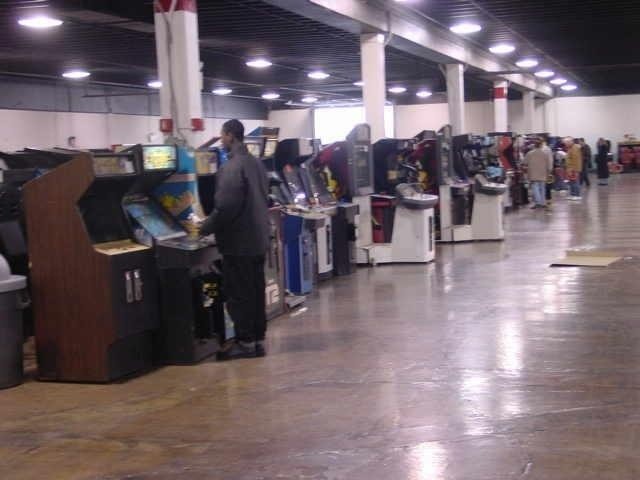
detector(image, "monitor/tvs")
[126,202,180,238]
[441,151,450,176]
[76,196,129,243]
[283,173,308,202]
[354,152,369,188]
[304,169,329,200]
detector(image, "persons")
[198,118,276,360]
[579,138,592,186]
[562,137,583,199]
[539,136,554,204]
[521,141,552,208]
[597,137,609,185]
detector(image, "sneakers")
[215,338,265,362]
